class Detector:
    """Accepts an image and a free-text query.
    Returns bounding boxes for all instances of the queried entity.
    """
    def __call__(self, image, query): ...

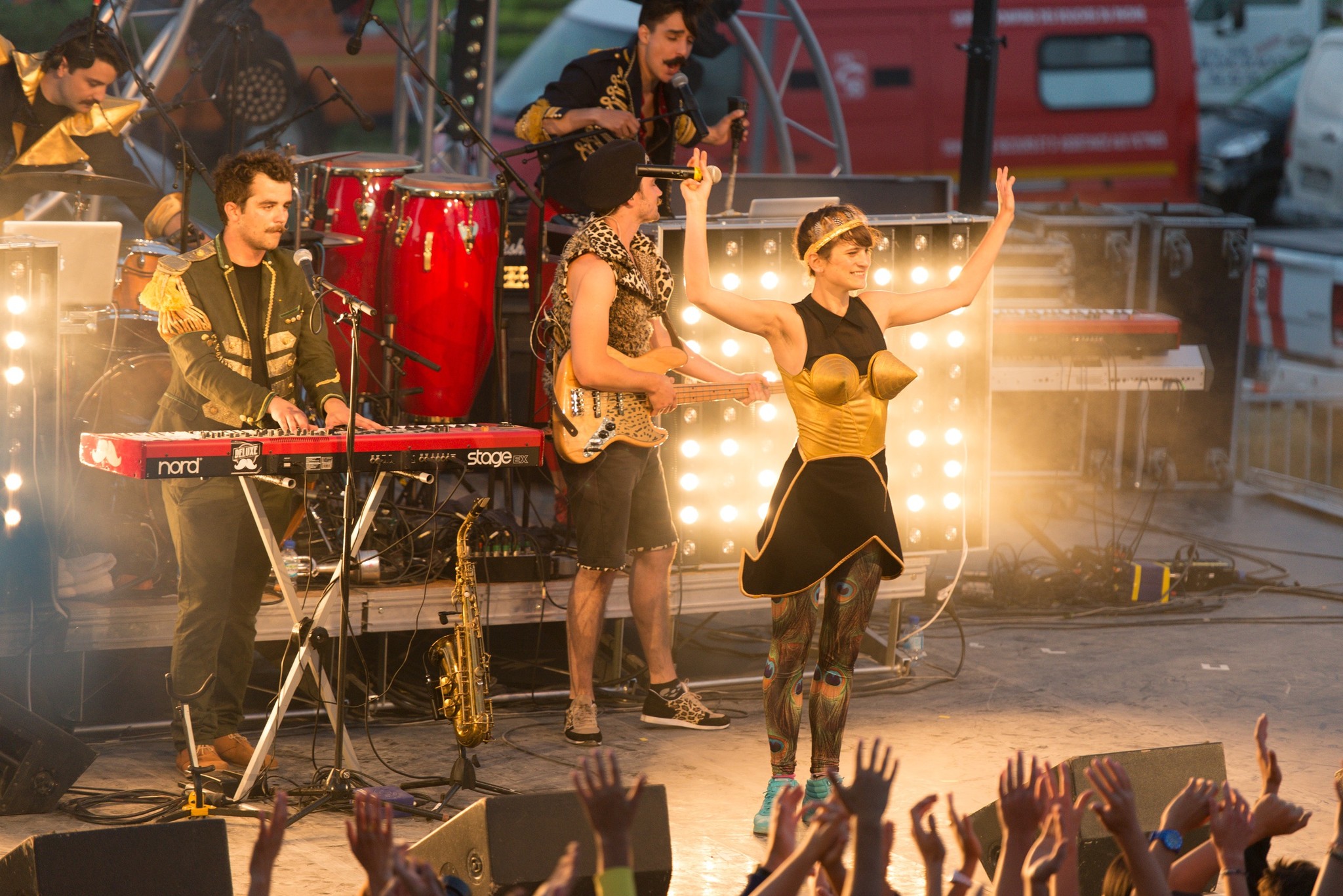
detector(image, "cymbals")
[279,227,364,250]
[285,149,363,167]
[1,169,164,199]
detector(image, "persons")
[1,21,204,256]
[245,712,1343,896]
[138,147,394,781]
[509,0,751,520]
[542,138,732,745]
[680,146,1017,832]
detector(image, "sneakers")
[175,744,229,777]
[564,695,603,746]
[640,679,730,730]
[801,772,845,822]
[214,733,279,770]
[753,779,799,834]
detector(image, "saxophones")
[424,493,498,750]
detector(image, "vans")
[408,1,1204,220]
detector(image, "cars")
[1194,46,1309,231]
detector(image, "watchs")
[948,869,972,887]
[1150,828,1183,854]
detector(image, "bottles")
[281,540,298,594]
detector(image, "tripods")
[254,264,516,827]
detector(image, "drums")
[70,352,320,550]
[313,157,427,401]
[85,237,187,354]
[378,169,502,425]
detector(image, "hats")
[578,139,647,210]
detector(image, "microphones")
[323,70,376,132]
[635,164,722,184]
[129,101,185,123]
[671,72,710,137]
[293,248,321,305]
[346,0,374,55]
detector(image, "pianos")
[76,422,550,803]
[995,302,1185,361]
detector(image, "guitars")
[552,341,788,465]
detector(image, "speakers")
[962,741,1231,896]
[1,691,100,813]
[0,817,233,896]
[405,783,673,895]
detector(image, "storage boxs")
[983,198,1256,492]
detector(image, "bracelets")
[1218,869,1247,876]
[1326,846,1343,862]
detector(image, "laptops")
[1,220,123,306]
